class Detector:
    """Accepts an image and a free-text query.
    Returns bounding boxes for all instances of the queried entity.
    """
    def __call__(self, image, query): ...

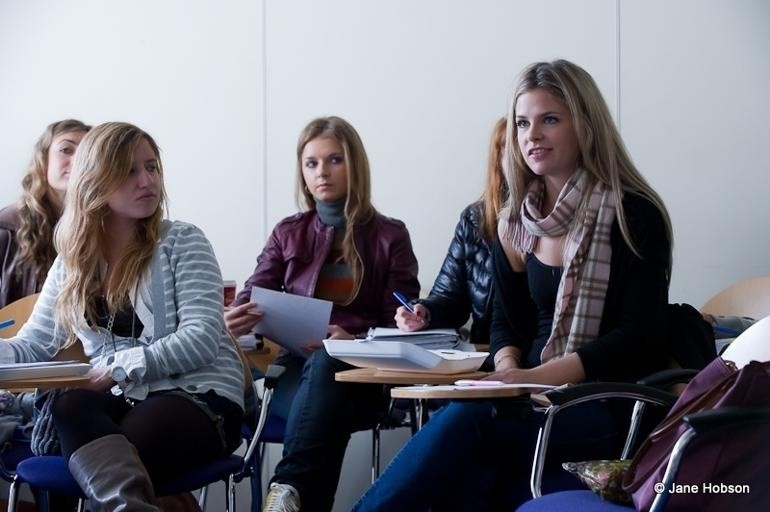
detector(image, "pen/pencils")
[223,306,262,317]
[712,326,740,336]
[0,319,15,329]
[393,291,424,323]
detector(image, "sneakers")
[260,481,302,512]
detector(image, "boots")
[67,433,164,512]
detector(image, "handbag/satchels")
[621,355,768,512]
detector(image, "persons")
[345,58,672,512]
[393,110,517,343]
[0,120,248,512]
[0,119,94,511]
[223,115,421,512]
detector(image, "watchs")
[109,362,130,391]
